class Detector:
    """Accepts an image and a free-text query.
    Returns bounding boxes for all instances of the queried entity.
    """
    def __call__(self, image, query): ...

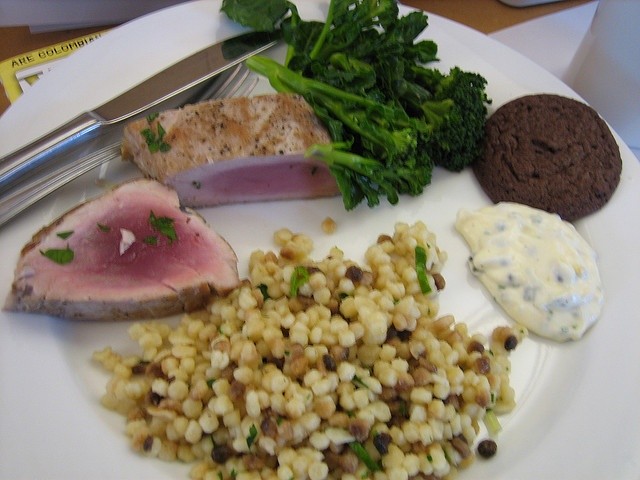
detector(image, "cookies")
[475,94,622,221]
[456,200,607,342]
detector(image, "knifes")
[1,31,281,194]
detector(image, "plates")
[0,1,639,480]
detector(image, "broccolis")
[220,0,490,210]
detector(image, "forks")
[0,64,259,224]
